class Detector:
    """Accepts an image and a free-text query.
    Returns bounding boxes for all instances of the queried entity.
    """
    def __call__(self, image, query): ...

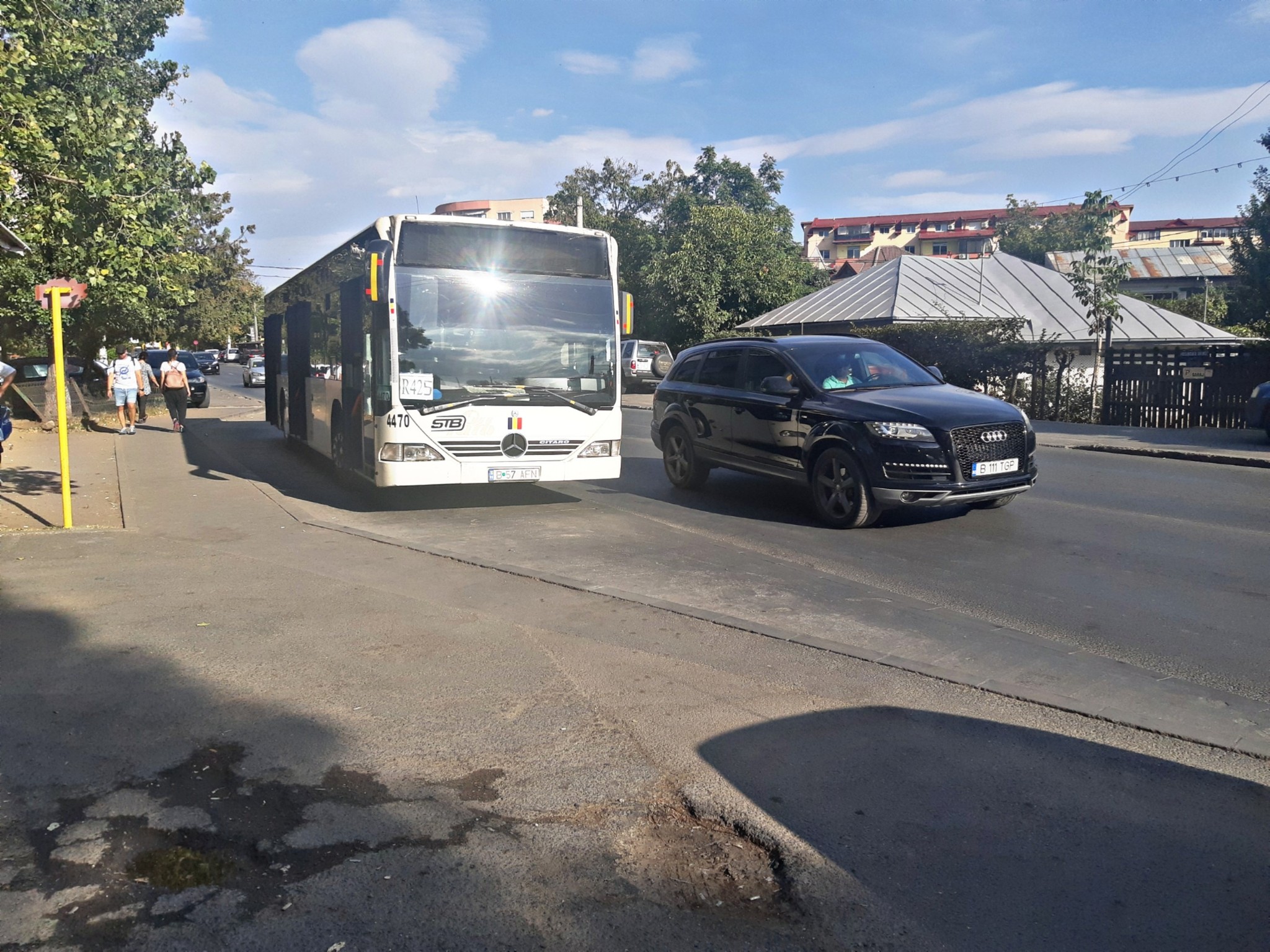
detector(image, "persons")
[822,360,863,389]
[160,349,191,432]
[107,344,145,435]
[0,361,16,486]
[335,360,342,380]
[137,352,159,424]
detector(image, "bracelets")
[107,389,111,390]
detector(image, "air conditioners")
[948,225,954,230]
[981,222,988,227]
[959,254,966,259]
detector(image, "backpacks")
[164,361,184,387]
[0,407,12,442]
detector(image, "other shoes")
[138,414,148,423]
[173,420,184,432]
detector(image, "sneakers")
[119,427,128,435]
[129,426,136,434]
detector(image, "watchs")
[139,388,144,391]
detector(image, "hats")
[115,344,126,354]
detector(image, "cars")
[242,358,266,388]
[311,364,342,380]
[0,357,108,410]
[192,352,220,375]
[1245,381,1270,438]
[205,350,224,361]
[145,350,210,408]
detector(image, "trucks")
[143,341,163,350]
[238,342,264,365]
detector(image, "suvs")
[650,333,1038,530]
[620,337,674,394]
[224,348,239,363]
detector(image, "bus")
[133,346,142,357]
[264,213,623,492]
